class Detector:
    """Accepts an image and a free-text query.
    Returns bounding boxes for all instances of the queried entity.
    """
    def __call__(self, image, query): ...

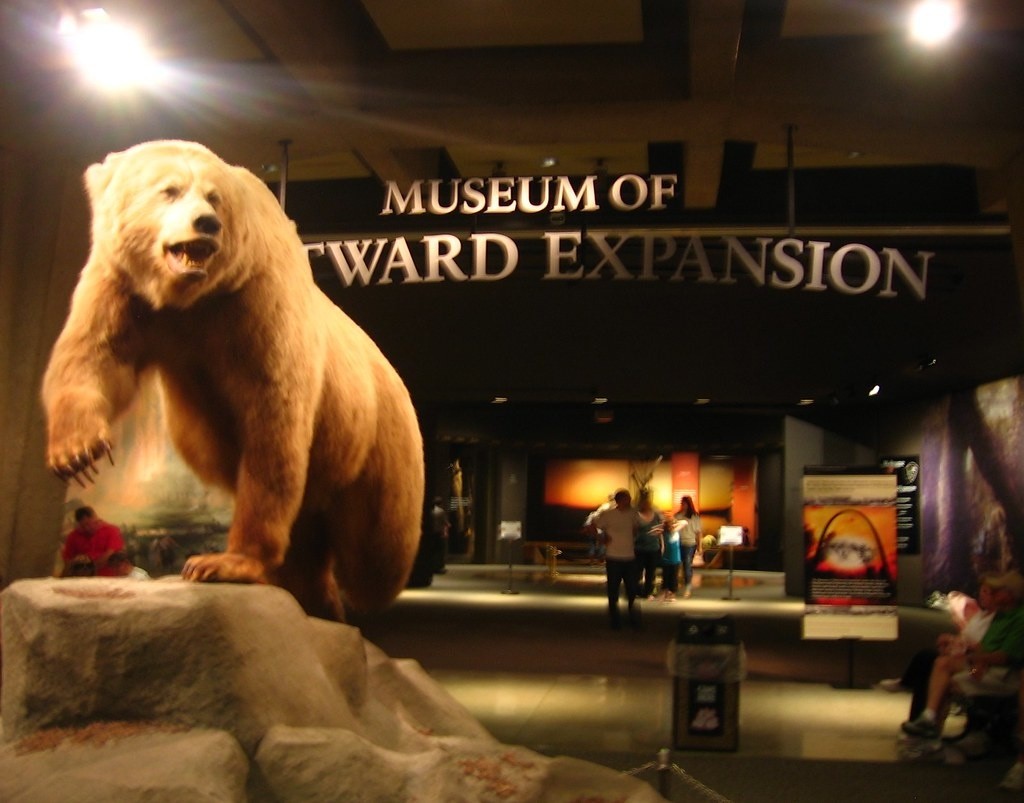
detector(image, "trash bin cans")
[666,618,742,753]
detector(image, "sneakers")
[905,739,941,754]
[901,717,936,739]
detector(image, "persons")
[108,551,151,580]
[656,511,688,599]
[66,554,95,576]
[61,506,127,576]
[902,570,1024,754]
[879,573,1000,743]
[585,487,646,629]
[631,487,665,600]
[673,496,701,598]
[431,496,450,575]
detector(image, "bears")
[39,138,424,625]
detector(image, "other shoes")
[879,677,905,691]
